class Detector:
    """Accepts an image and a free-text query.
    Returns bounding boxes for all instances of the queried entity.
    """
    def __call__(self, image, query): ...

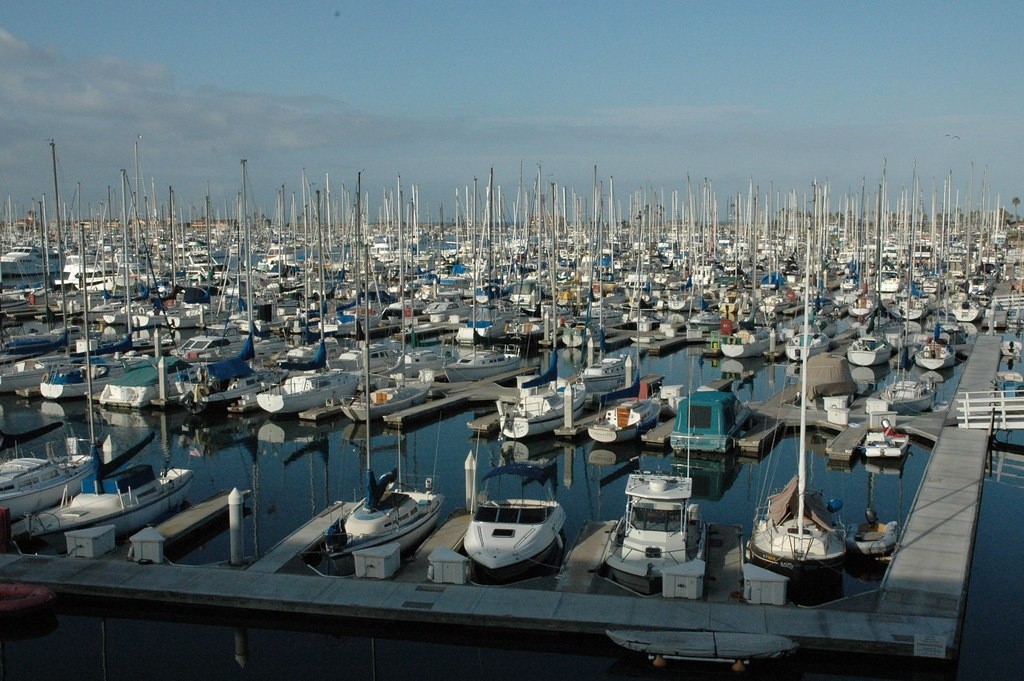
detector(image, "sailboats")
[323,243,446,577]
[25,223,194,550]
[1,142,1011,453]
[745,228,848,580]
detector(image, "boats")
[865,452,908,475]
[462,460,565,579]
[605,630,800,661]
[0,438,95,521]
[861,432,909,457]
[999,341,1022,357]
[1000,355,1022,369]
[603,469,707,595]
[994,372,1023,388]
[846,508,899,557]
